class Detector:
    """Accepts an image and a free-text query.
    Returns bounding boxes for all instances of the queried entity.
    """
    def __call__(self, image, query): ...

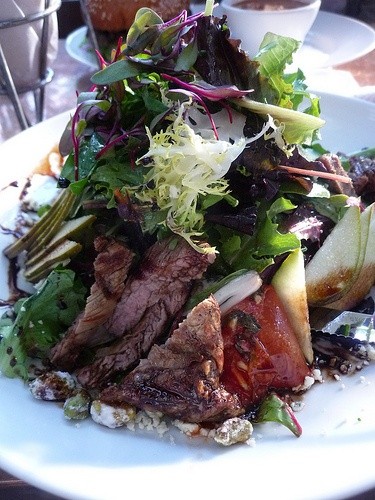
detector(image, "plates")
[0,86,375,500]
[66,11,374,86]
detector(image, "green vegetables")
[0,1,375,438]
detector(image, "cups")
[212,0,323,59]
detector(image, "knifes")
[301,306,374,343]
